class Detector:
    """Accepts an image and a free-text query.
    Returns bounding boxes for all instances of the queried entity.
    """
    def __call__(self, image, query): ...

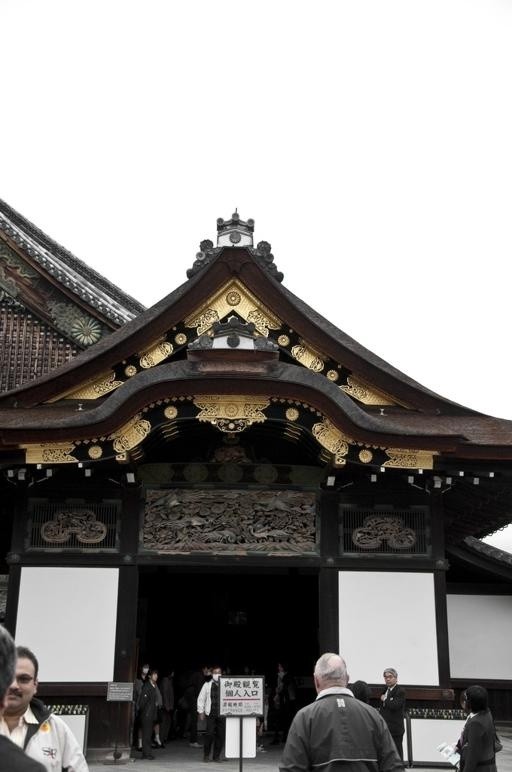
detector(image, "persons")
[378,667,406,761]
[454,691,471,754]
[349,680,372,703]
[460,686,497,772]
[0,625,48,772]
[0,646,89,772]
[278,652,405,772]
[131,659,295,763]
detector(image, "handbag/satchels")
[494,742,502,752]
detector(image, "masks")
[212,673,222,681]
[142,668,149,674]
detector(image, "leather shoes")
[189,742,204,747]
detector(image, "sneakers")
[204,759,222,762]
[135,740,166,751]
[142,754,154,759]
